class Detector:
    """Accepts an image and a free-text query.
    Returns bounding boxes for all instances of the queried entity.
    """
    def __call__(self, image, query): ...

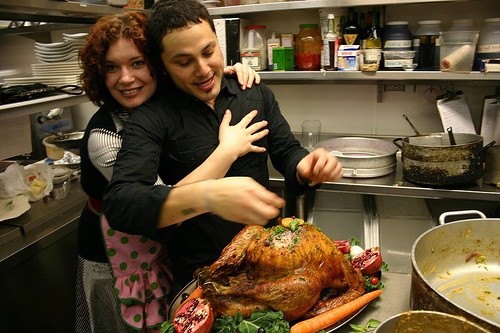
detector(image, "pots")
[409,210,500,333]
[393,133,496,189]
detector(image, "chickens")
[197,224,366,319]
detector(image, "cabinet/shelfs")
[201,0,500,202]
[0,0,149,273]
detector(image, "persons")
[73,9,270,333]
[102,0,343,291]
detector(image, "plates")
[0,196,32,222]
[0,33,90,91]
[200,0,221,8]
[166,277,369,333]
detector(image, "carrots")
[176,286,203,313]
[290,289,384,333]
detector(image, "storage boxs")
[271,46,294,68]
[338,44,361,70]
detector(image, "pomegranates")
[172,297,213,333]
[333,240,350,254]
[351,246,382,275]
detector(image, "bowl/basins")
[373,311,491,333]
[313,136,399,179]
[43,131,85,161]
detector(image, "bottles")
[476,18,500,71]
[267,31,281,71]
[295,24,323,71]
[239,24,267,72]
[412,19,443,71]
[445,18,479,32]
[383,20,411,70]
[321,4,386,71]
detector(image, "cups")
[438,31,481,74]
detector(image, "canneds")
[241,24,268,71]
[295,23,323,70]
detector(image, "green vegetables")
[160,305,290,333]
[254,218,323,249]
[342,236,390,292]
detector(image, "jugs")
[302,119,321,151]
